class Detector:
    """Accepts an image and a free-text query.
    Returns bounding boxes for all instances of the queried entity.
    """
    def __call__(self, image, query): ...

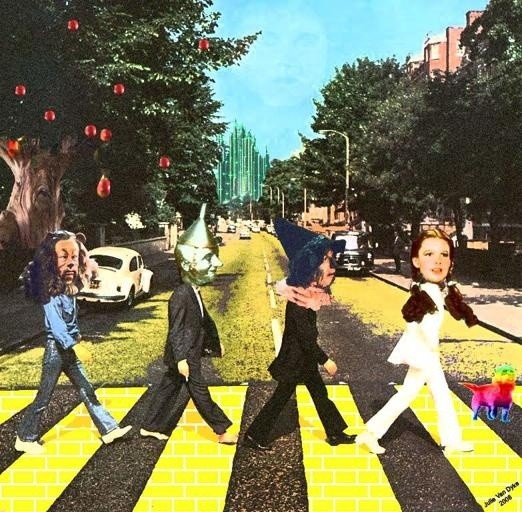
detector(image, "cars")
[331,230,376,276]
[244,218,278,237]
[239,230,251,239]
[228,224,237,233]
[71,244,156,311]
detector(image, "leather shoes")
[325,432,358,447]
[354,432,386,454]
[139,427,169,441]
[216,431,272,450]
[445,441,475,453]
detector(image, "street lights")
[318,128,352,231]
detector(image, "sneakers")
[101,424,132,444]
[12,435,46,455]
